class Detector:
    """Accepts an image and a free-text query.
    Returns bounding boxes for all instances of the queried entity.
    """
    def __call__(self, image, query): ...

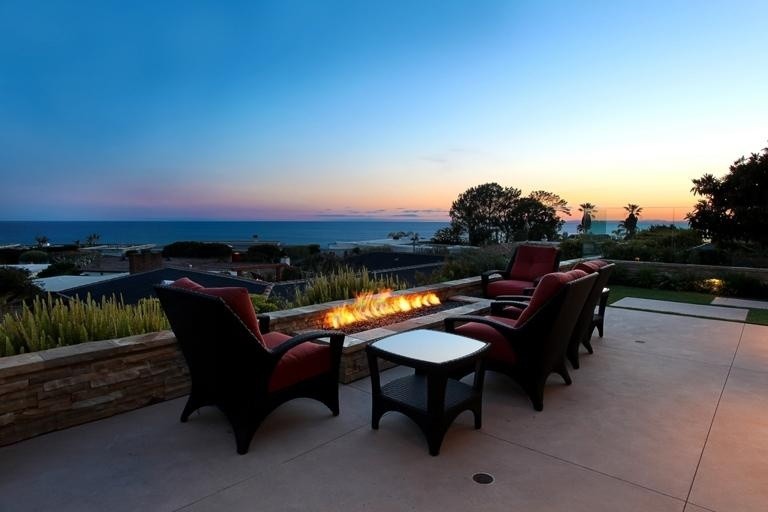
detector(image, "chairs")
[442,240,614,413]
[150,275,346,457]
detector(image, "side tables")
[367,327,493,457]
